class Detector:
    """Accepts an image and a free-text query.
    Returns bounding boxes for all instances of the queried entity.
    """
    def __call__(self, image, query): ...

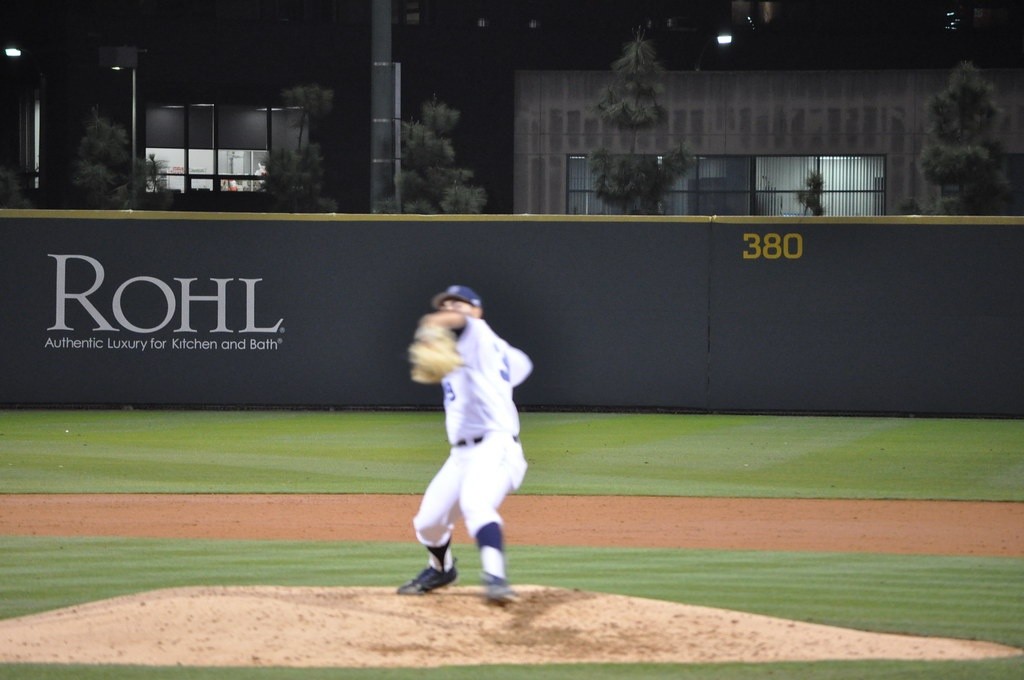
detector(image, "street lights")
[111,66,138,210]
[3,47,40,191]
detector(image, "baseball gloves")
[406,324,462,385]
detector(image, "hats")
[431,284,483,309]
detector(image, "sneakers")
[488,584,524,604]
[399,566,458,596]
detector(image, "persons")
[397,286,534,609]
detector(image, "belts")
[456,434,524,445]
